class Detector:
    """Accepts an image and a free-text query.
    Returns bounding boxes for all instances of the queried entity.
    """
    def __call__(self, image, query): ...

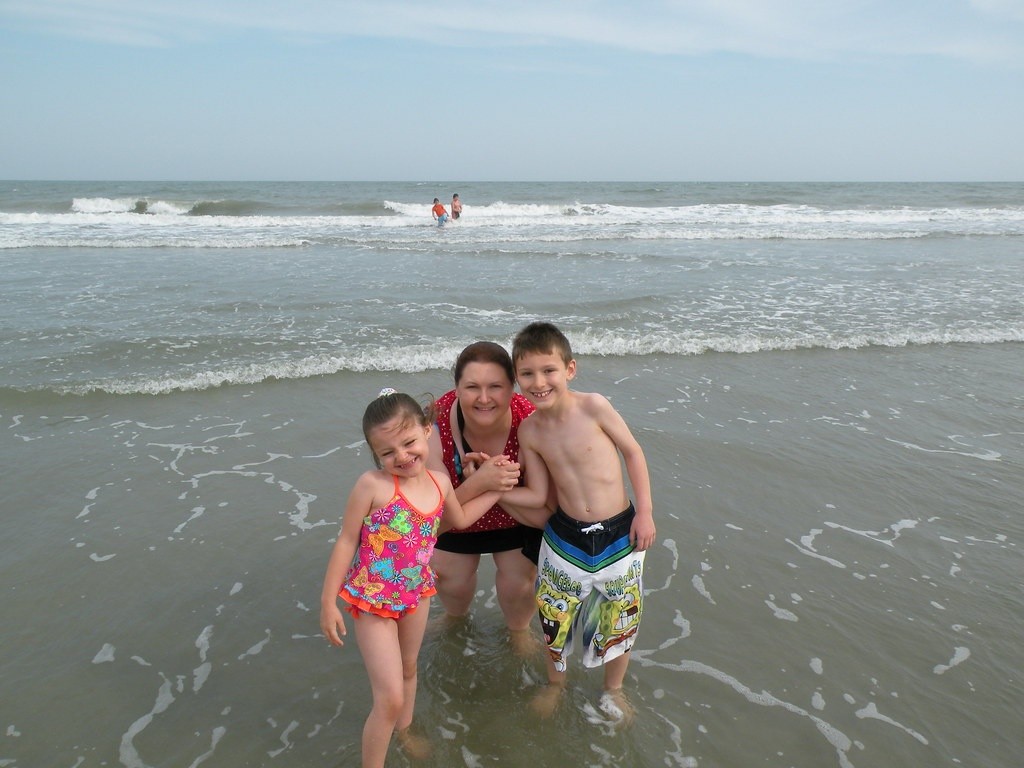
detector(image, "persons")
[423,341,559,630]
[463,320,656,689]
[319,387,514,768]
[432,198,449,226]
[451,193,462,220]
